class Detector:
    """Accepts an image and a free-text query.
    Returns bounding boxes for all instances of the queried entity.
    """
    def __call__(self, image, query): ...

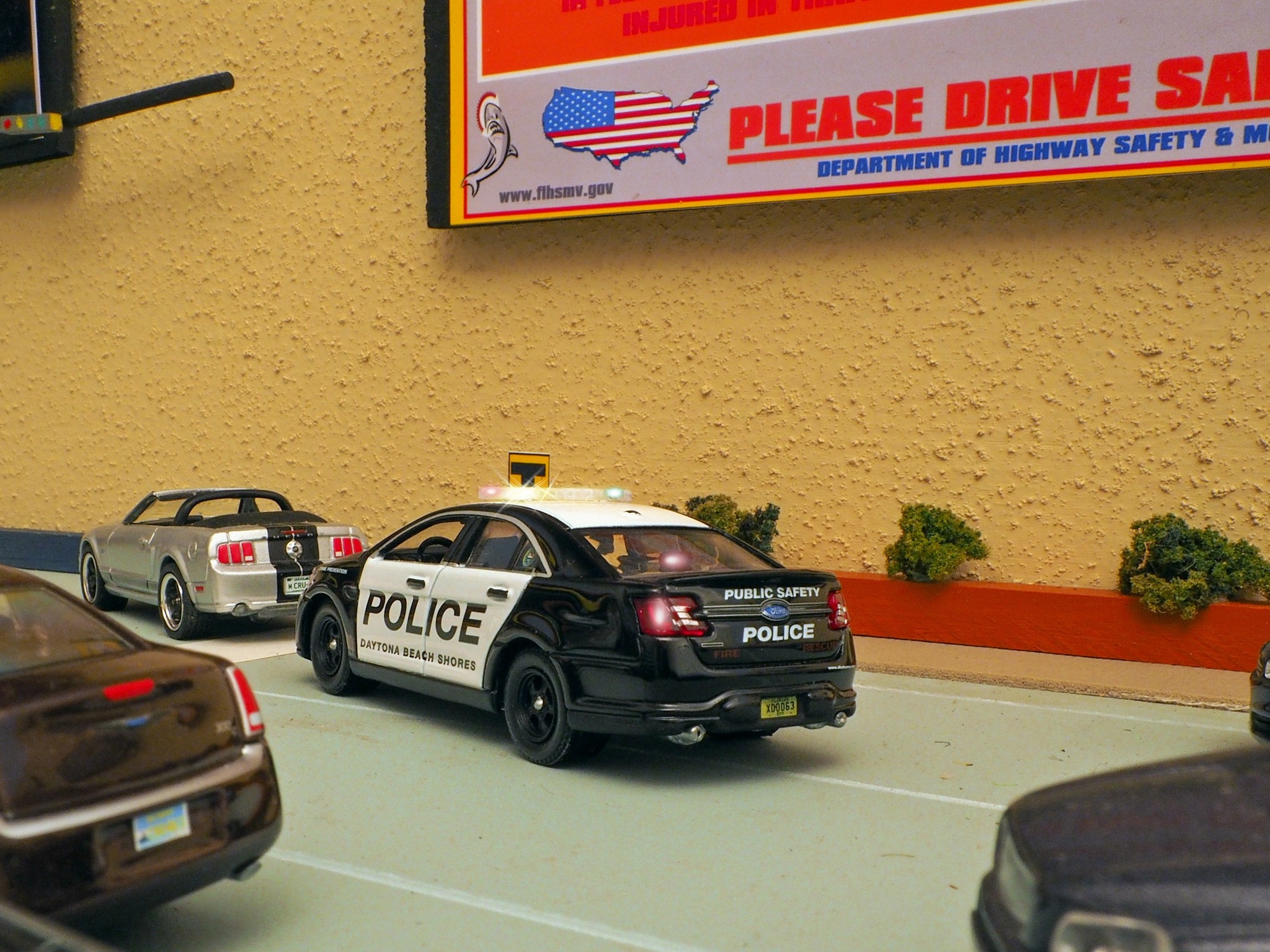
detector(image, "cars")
[1249,639,1270,743]
[296,486,857,768]
[0,563,283,939]
[968,744,1270,952]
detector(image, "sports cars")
[77,489,367,642]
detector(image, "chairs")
[472,536,523,570]
